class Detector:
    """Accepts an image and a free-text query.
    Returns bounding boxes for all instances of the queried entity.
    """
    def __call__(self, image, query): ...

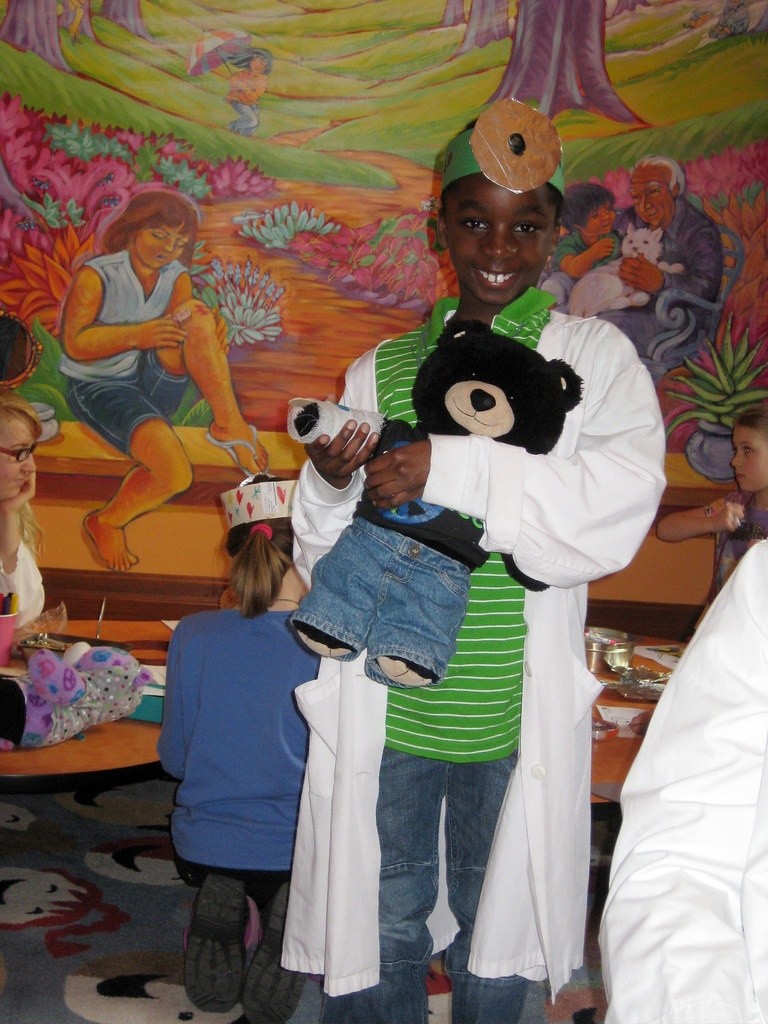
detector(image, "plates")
[611,668,672,684]
[617,683,666,699]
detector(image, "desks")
[586,637,689,819]
[0,620,173,792]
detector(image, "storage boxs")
[18,632,133,668]
[584,626,635,673]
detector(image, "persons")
[160,474,318,1024]
[281,96,667,1024]
[598,536,768,1024]
[0,391,45,630]
[658,405,768,629]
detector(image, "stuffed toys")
[287,320,581,683]
[0,641,151,750]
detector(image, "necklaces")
[277,598,301,607]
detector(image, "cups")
[0,611,20,667]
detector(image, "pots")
[584,626,635,674]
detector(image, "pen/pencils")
[0,591,20,616]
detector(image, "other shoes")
[180,870,248,1013]
[240,880,310,1023]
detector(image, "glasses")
[0,442,38,462]
